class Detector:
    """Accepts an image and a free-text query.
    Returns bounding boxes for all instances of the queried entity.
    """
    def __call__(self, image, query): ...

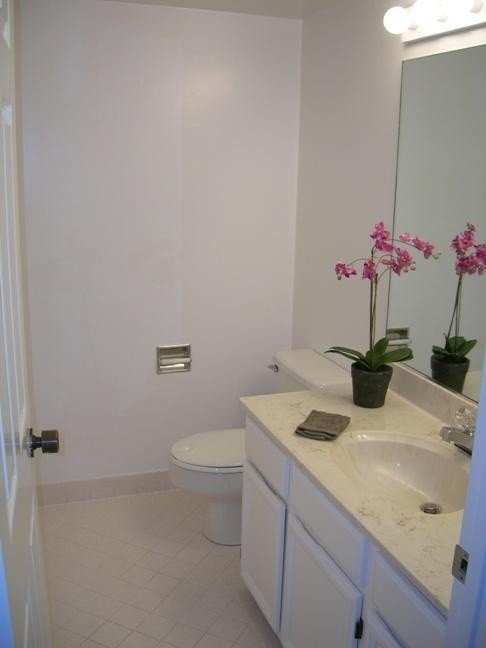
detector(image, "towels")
[294,409,351,441]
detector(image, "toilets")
[170,347,353,545]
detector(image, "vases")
[352,362,392,408]
[431,355,470,393]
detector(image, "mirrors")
[386,45,486,404]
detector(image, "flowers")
[431,222,486,364]
[324,221,443,372]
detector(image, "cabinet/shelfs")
[239,414,369,648]
[367,549,448,648]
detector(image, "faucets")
[440,405,474,454]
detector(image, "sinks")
[329,428,471,520]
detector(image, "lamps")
[383,0,486,44]
